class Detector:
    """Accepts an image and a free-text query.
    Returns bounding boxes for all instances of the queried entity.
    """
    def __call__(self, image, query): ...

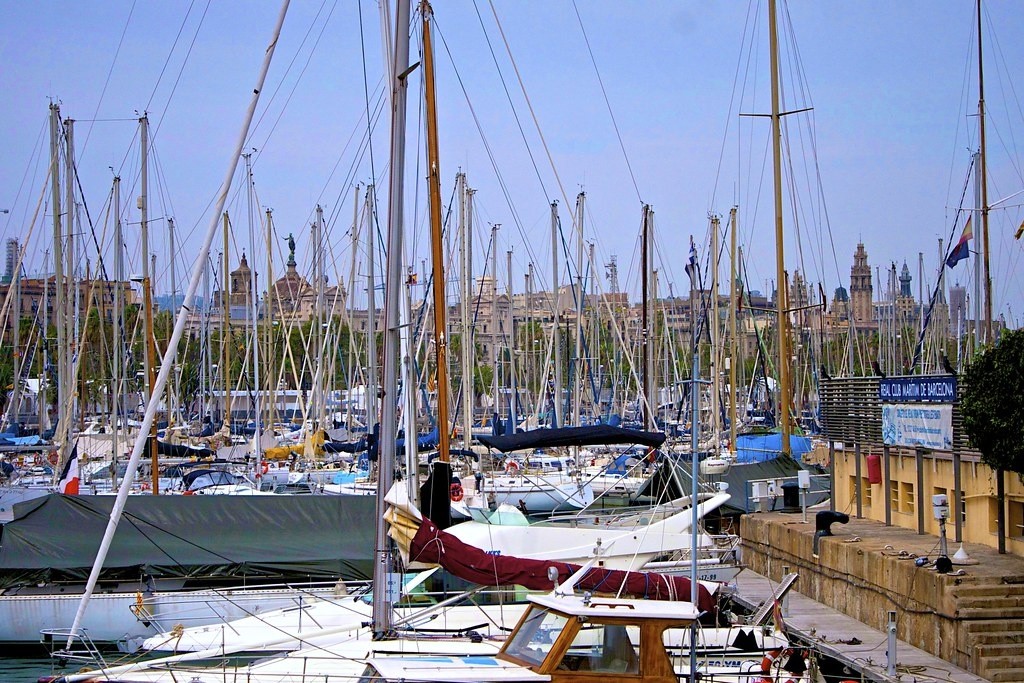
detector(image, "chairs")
[608,658,628,672]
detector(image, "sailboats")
[0,1,1024,683]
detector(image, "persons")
[281,233,295,254]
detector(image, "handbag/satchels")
[936,555,953,573]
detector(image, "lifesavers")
[254,461,268,474]
[183,491,197,495]
[451,484,464,502]
[760,649,803,683]
[506,462,518,471]
[33,454,42,465]
[48,453,59,465]
[141,482,149,491]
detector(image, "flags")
[427,370,435,392]
[408,272,418,285]
[1013,219,1024,241]
[944,214,973,269]
[450,426,462,439]
[57,445,79,495]
[685,243,695,286]
